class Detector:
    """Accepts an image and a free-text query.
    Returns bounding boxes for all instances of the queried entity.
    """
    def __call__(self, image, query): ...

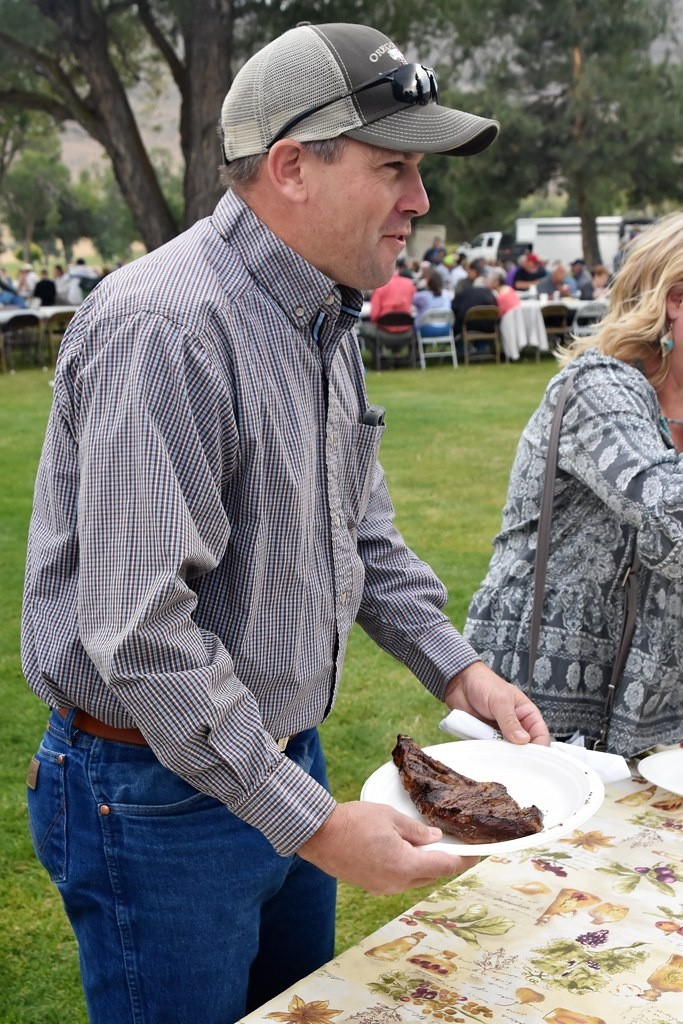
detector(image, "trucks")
[455,213,655,280]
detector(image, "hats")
[221,21,501,165]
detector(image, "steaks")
[391,734,544,843]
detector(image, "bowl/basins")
[637,749,683,801]
[360,740,604,856]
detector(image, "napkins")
[438,709,632,784]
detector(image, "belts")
[60,706,146,746]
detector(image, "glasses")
[266,63,440,148]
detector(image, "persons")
[21,18,551,1024]
[0,229,646,365]
[459,209,683,765]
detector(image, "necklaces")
[634,360,683,436]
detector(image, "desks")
[360,290,593,316]
[233,740,683,1024]
[0,305,81,325]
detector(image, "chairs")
[462,305,502,365]
[500,304,549,365]
[415,308,458,369]
[3,315,42,374]
[45,312,75,364]
[568,300,607,338]
[374,312,417,371]
[535,305,570,362]
